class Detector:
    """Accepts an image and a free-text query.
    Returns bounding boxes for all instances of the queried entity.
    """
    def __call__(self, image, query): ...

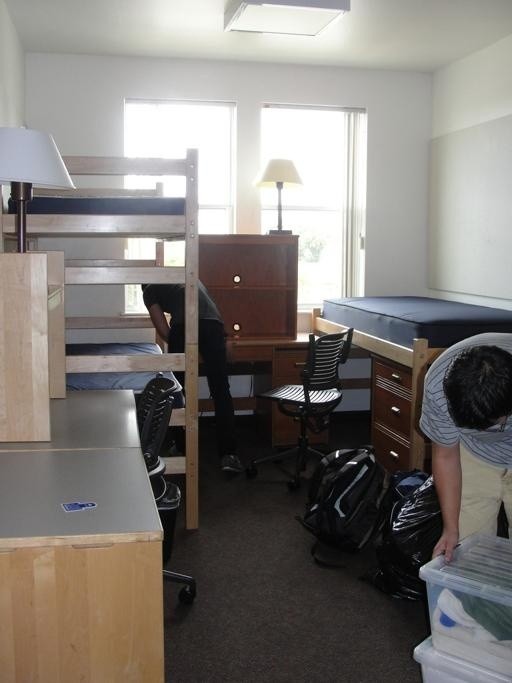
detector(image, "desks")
[2,450,169,680]
[172,327,328,446]
[2,386,141,452]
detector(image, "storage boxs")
[419,526,511,672]
[413,633,512,682]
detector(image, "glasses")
[483,416,508,432]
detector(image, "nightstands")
[1,249,71,446]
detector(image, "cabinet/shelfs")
[369,357,414,478]
[199,236,297,339]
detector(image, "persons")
[418,331,512,564]
[141,278,248,475]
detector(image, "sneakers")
[222,455,244,473]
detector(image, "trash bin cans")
[152,481,181,562]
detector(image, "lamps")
[254,158,305,232]
[222,0,349,39]
[0,125,76,252]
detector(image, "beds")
[1,149,206,533]
[310,293,511,488]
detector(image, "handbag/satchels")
[295,449,389,549]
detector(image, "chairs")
[130,369,197,614]
[246,328,355,483]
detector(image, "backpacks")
[361,468,443,601]
